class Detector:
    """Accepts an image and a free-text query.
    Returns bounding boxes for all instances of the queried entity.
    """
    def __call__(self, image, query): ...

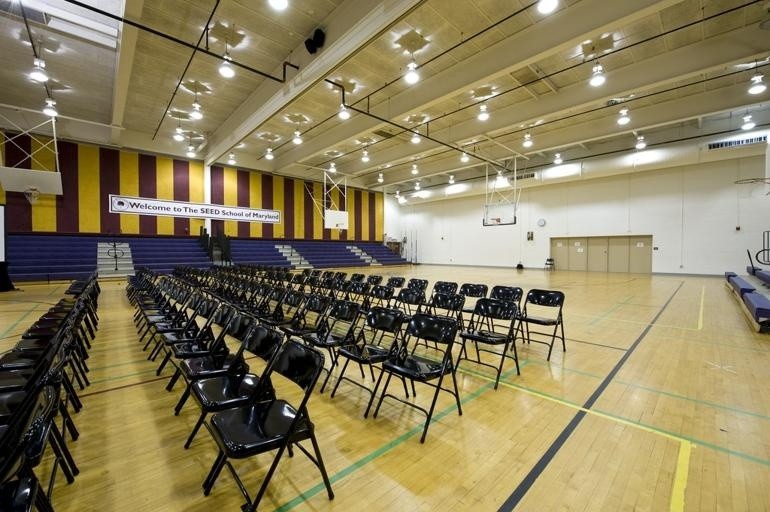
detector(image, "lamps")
[635,133,646,150]
[43,87,58,117]
[190,88,202,118]
[748,60,767,94]
[329,162,337,174]
[411,130,422,143]
[30,41,48,82]
[377,171,384,183]
[521,132,533,147]
[186,136,195,157]
[478,105,490,121]
[292,129,302,144]
[394,189,400,198]
[265,146,274,160]
[174,112,184,141]
[405,41,419,84]
[495,170,503,182]
[741,111,755,130]
[411,164,418,175]
[361,148,370,162]
[553,151,562,165]
[415,182,420,190]
[616,105,631,126]
[460,152,469,163]
[339,102,349,120]
[590,48,604,86]
[228,152,236,164]
[449,174,455,185]
[219,38,234,78]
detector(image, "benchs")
[725,266,770,333]
[230,239,411,269]
[7,236,212,280]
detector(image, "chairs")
[127,268,525,416]
[373,314,462,444]
[203,338,335,512]
[544,259,555,271]
[510,288,567,361]
[185,325,285,484]
[0,273,100,512]
